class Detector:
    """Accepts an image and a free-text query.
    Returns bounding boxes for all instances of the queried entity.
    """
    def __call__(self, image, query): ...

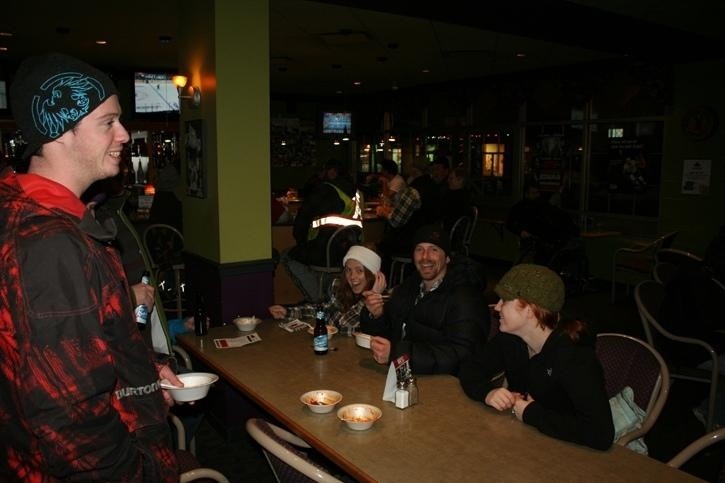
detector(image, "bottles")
[192,293,208,337]
[313,303,329,355]
[394,378,419,410]
[133,270,152,331]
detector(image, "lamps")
[172,73,202,109]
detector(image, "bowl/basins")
[353,331,371,350]
[298,389,384,431]
[308,326,338,339]
[233,316,263,332]
[159,370,220,402]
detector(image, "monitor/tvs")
[132,67,180,114]
[323,112,352,134]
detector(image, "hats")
[494,262,566,314]
[413,222,454,259]
[9,49,120,160]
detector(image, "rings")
[512,410,515,413]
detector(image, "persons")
[82,153,172,357]
[271,159,579,270]
[0,53,196,483]
[360,224,491,375]
[268,242,393,337]
[458,264,614,451]
